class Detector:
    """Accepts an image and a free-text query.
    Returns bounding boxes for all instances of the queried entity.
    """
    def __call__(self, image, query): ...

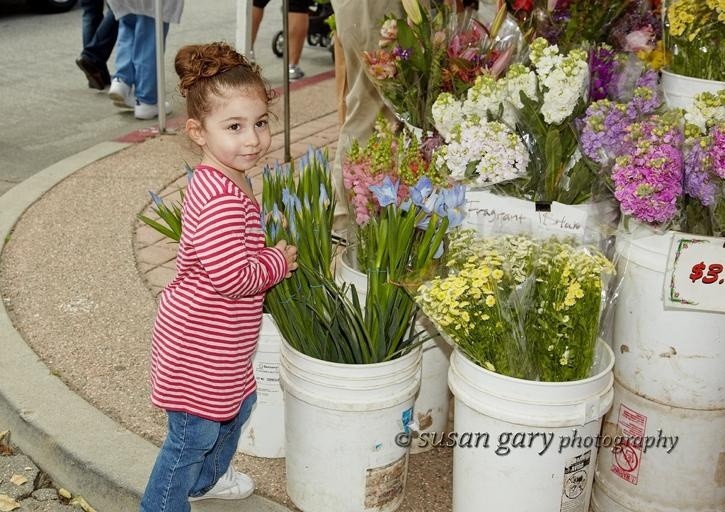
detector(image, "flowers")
[135,147,286,316]
[429,38,613,202]
[268,149,449,365]
[418,231,626,380]
[361,1,516,130]
[342,118,444,271]
[664,1,725,78]
[579,69,725,238]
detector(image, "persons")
[329,0,402,246]
[139,41,299,512]
[250,0,310,79]
[75,0,119,89]
[107,0,184,121]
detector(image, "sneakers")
[188,465,255,502]
[249,52,256,65]
[289,67,306,83]
[76,54,173,121]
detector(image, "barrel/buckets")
[612,213,725,411]
[659,65,725,135]
[278,321,423,512]
[588,379,725,512]
[336,243,453,455]
[446,337,616,512]
[236,313,304,482]
[552,199,620,261]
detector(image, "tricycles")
[271,2,334,58]
